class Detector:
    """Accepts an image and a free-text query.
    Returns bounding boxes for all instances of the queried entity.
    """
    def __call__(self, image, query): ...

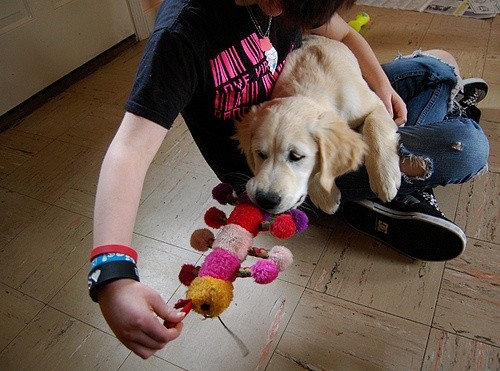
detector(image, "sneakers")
[447,78,488,118]
[341,186,467,261]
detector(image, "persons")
[88,0,490,360]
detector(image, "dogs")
[231,35,402,215]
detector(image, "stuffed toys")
[163,183,308,330]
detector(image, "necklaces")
[243,0,273,52]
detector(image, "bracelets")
[87,260,140,303]
[90,253,136,271]
[88,244,138,263]
